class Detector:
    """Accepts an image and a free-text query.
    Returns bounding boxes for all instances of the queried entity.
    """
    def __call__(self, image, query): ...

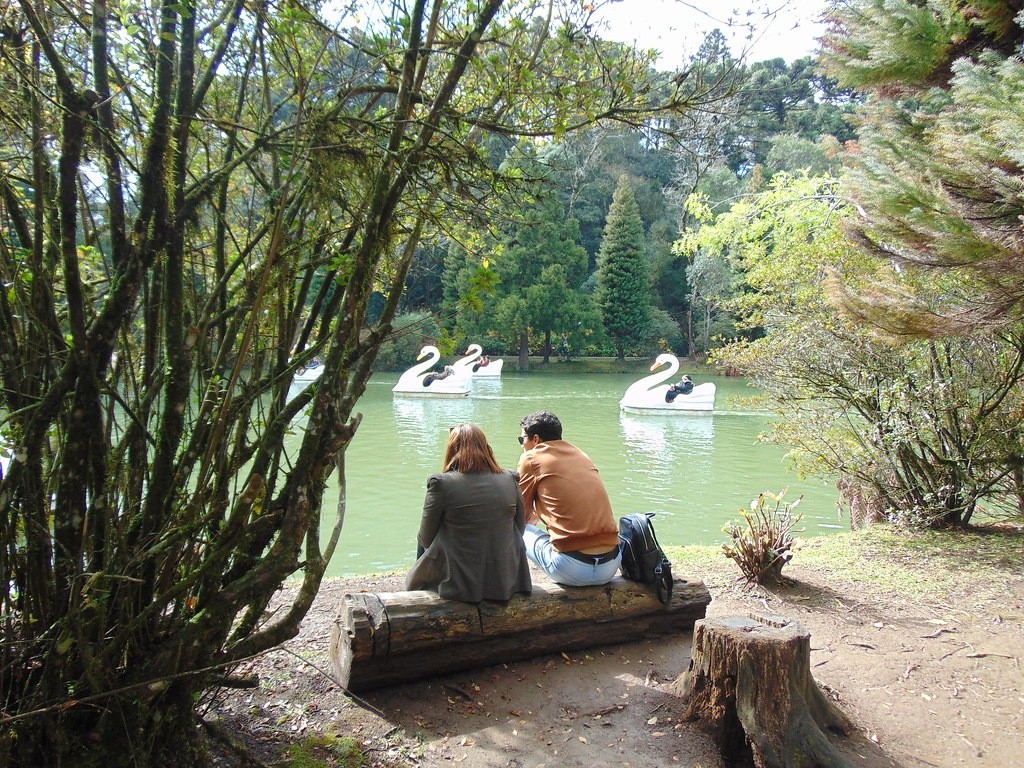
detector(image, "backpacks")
[618,513,673,607]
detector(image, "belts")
[563,545,620,565]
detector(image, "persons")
[666,375,694,400]
[300,357,321,374]
[517,413,622,586]
[423,366,449,387]
[403,422,535,604]
[473,355,489,373]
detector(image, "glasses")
[518,436,527,445]
[450,428,454,433]
